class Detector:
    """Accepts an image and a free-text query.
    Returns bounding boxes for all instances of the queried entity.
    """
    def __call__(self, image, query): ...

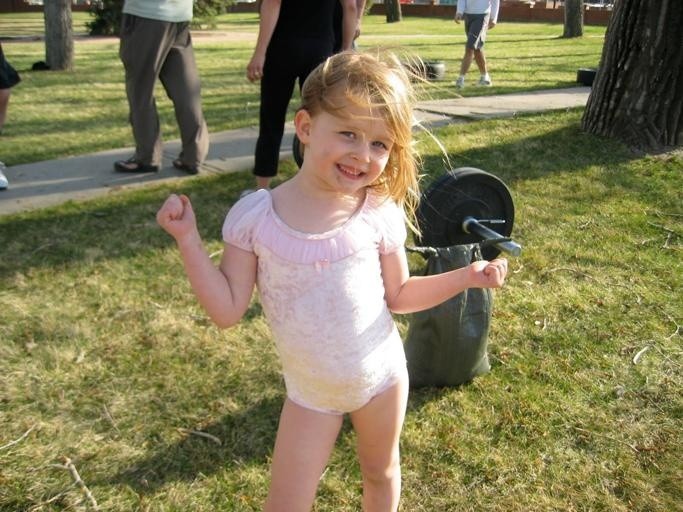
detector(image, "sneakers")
[115,158,159,173]
[0,170,8,189]
[456,77,465,88]
[173,158,200,174]
[480,79,491,86]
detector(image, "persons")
[0,45,24,192]
[153,47,510,512]
[453,1,499,89]
[110,0,210,175]
[351,1,367,51]
[240,1,359,199]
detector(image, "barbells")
[292,135,522,258]
